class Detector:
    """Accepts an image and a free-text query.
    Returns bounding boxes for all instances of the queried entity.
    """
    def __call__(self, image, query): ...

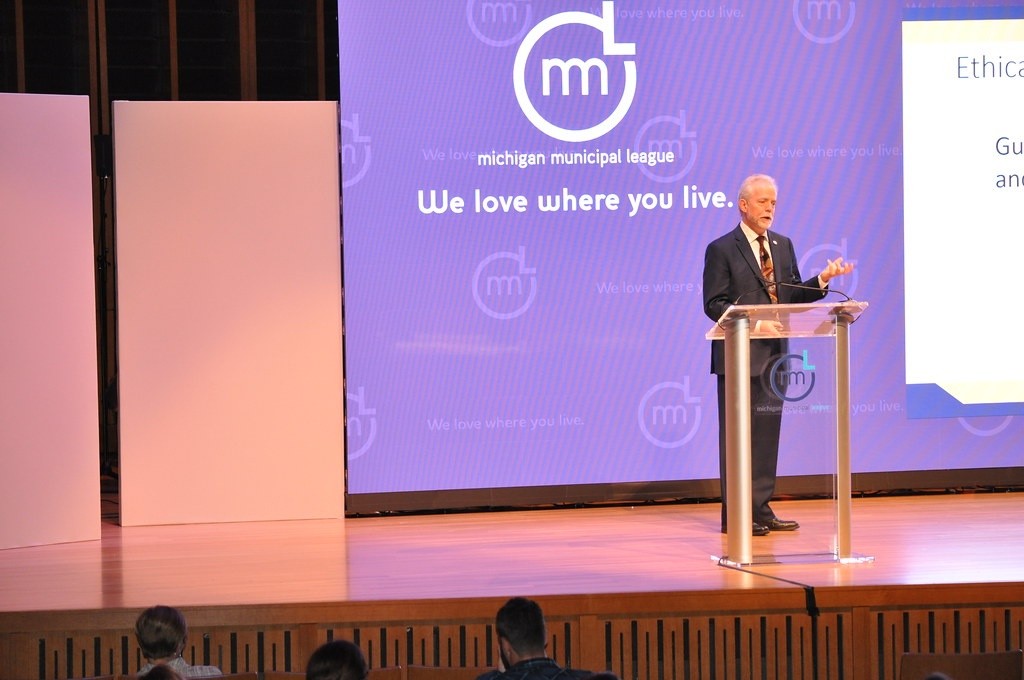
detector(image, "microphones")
[732,273,853,305]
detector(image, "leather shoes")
[764,517,800,531]
[721,521,769,535]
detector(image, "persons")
[306,640,369,680]
[136,605,223,680]
[490,597,594,680]
[703,174,857,536]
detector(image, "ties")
[756,236,778,304]
